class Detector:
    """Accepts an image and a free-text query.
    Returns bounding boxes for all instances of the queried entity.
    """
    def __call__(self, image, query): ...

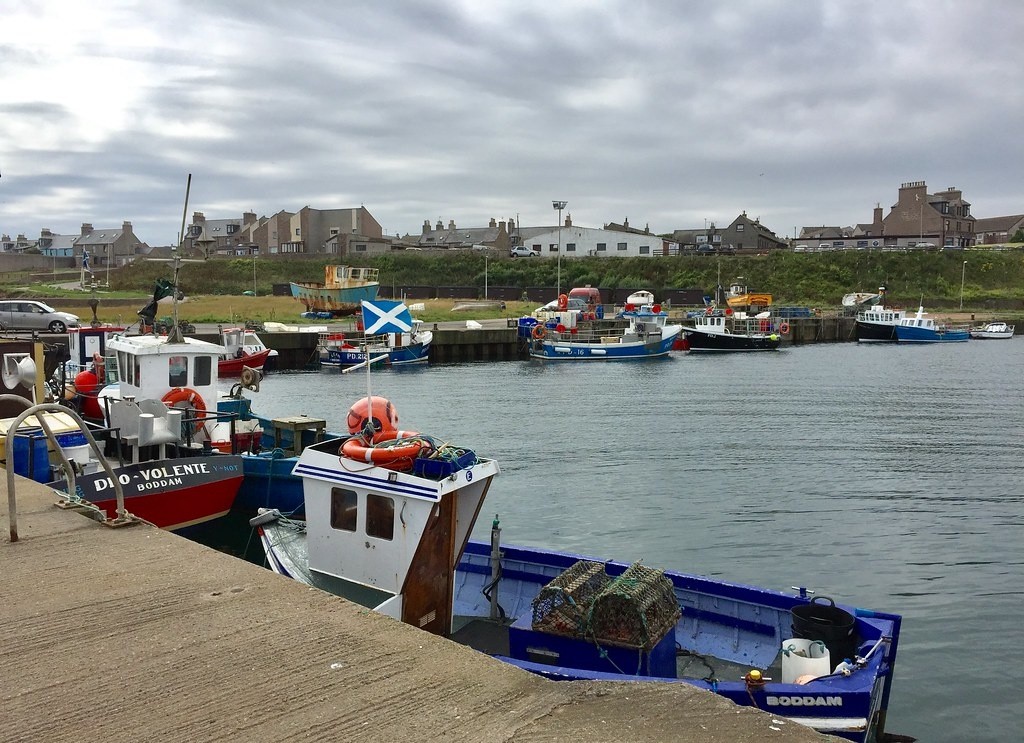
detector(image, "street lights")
[959,260,968,310]
[553,199,569,296]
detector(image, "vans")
[569,287,601,316]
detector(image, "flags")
[83,250,89,270]
[362,299,413,334]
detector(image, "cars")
[718,244,736,256]
[693,244,716,257]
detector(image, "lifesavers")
[557,294,567,307]
[162,387,206,437]
[360,437,433,473]
[344,430,424,459]
[532,324,547,338]
[779,322,789,334]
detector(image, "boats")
[968,317,1016,339]
[891,304,970,343]
[676,307,791,355]
[286,261,380,316]
[57,265,353,527]
[850,302,906,344]
[842,292,881,315]
[524,304,683,362]
[701,282,773,309]
[238,394,904,742]
[0,355,244,535]
[199,325,272,378]
[306,316,433,375]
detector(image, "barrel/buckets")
[791,596,855,675]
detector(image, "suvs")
[0,300,80,335]
[533,298,589,317]
[792,241,963,256]
[510,245,540,258]
